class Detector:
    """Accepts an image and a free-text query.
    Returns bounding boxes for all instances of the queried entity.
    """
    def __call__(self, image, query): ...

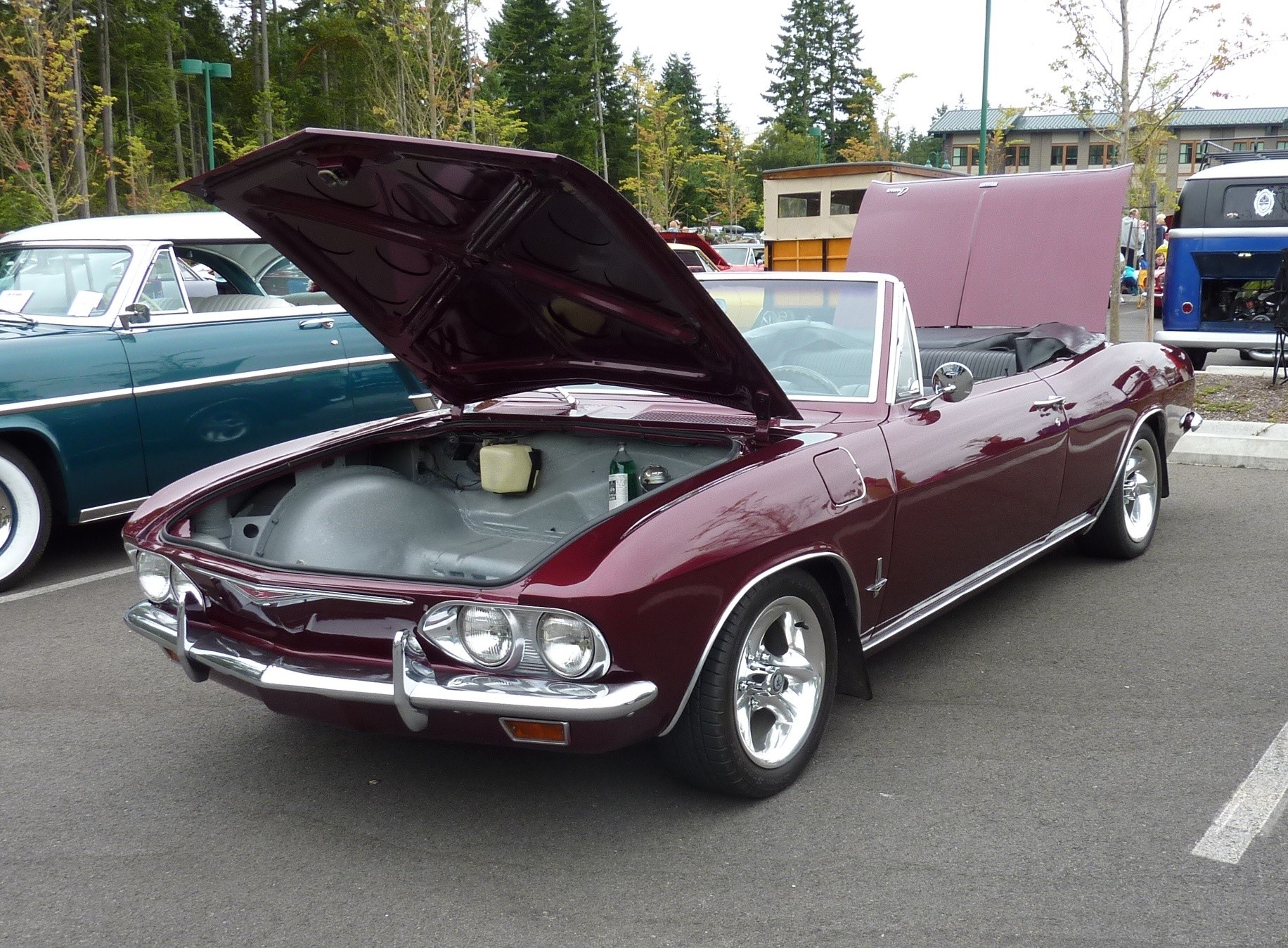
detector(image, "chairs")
[136,291,340,313]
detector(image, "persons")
[1143,213,1169,260]
[1138,220,1146,255]
[1120,266,1137,292]
[1120,208,1140,268]
[682,227,689,233]
[646,218,662,232]
[669,220,681,232]
[1155,253,1166,269]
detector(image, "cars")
[0,252,219,310]
[0,212,438,598]
[656,224,767,274]
[1152,269,1165,319]
[120,127,1204,804]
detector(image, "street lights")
[181,59,231,212]
[925,151,951,170]
[807,126,821,165]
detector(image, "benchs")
[777,344,1017,398]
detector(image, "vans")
[1152,140,1288,371]
